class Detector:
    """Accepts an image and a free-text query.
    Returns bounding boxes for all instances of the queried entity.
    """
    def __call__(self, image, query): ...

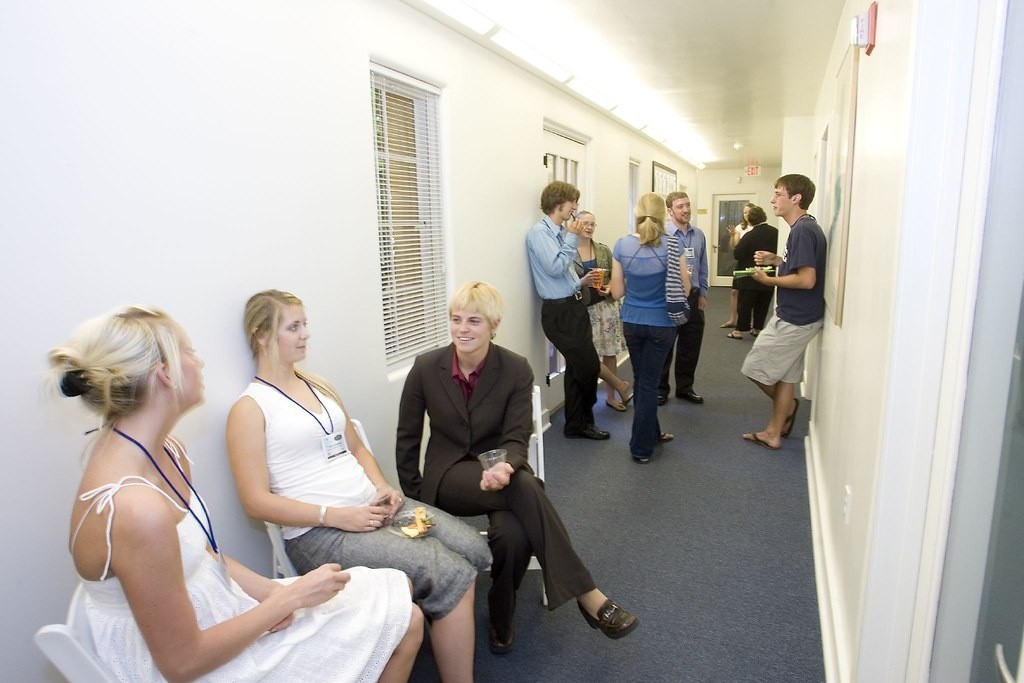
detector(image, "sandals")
[635,456,649,463]
[656,433,673,443]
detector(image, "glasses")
[583,221,598,227]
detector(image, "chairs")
[475,385,549,606]
[35,584,123,683]
[265,419,374,580]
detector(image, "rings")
[368,520,374,527]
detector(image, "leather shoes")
[565,425,610,440]
[577,597,639,639]
[676,389,704,403]
[656,395,667,404]
[489,622,514,654]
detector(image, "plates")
[382,508,439,538]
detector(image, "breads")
[401,507,428,538]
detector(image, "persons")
[575,211,633,412]
[525,180,612,449]
[225,289,493,683]
[48,304,424,683]
[609,191,692,464]
[740,174,828,452]
[395,282,640,653]
[719,202,778,340]
[657,191,705,406]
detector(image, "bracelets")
[319,505,327,529]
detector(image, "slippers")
[750,329,758,336]
[727,332,742,339]
[780,398,798,436]
[720,322,736,328]
[743,433,773,448]
[623,393,634,403]
[606,400,626,411]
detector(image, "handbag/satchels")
[665,238,690,324]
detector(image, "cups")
[687,265,692,278]
[364,483,393,520]
[477,449,508,483]
[592,268,605,289]
